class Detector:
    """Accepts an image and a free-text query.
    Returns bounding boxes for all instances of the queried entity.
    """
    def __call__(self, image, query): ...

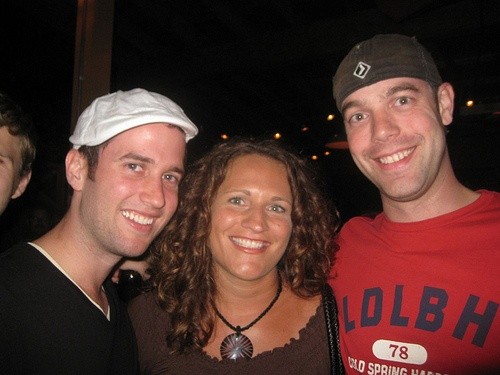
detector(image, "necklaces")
[210,271,283,360]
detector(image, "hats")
[69,88,198,150]
[333,34,442,112]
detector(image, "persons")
[0,88,200,375]
[108,33,500,375]
[0,92,61,265]
[96,139,344,375]
